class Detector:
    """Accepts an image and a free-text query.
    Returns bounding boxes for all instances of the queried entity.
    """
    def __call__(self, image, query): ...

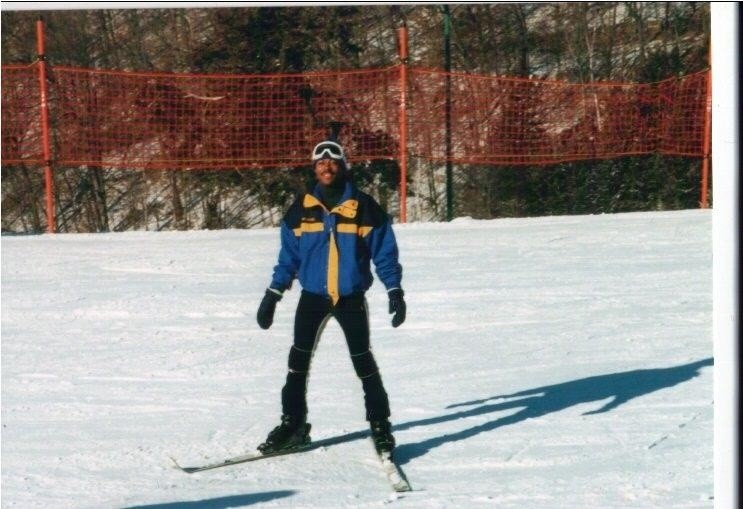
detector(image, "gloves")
[386,287,405,328]
[255,287,282,330]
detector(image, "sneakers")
[370,418,394,452]
[265,413,311,450]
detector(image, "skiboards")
[170,429,411,493]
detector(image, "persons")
[256,138,406,456]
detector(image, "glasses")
[312,141,345,162]
